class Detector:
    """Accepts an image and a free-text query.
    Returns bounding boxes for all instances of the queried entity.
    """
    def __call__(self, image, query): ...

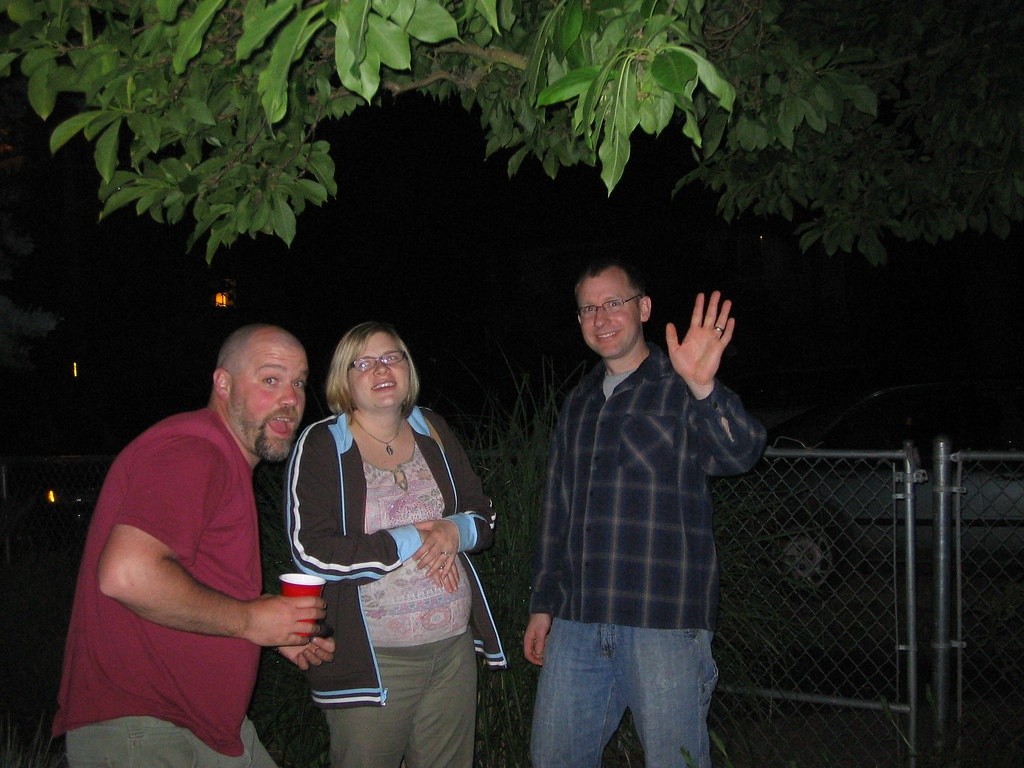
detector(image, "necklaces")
[355,418,399,455]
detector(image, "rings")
[714,327,724,332]
[440,567,444,569]
[441,552,450,556]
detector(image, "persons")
[283,323,505,768]
[51,324,336,767]
[524,259,767,768]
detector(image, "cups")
[279,573,327,639]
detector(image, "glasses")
[577,294,643,316]
[346,352,407,372]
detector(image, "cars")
[713,381,1024,552]
